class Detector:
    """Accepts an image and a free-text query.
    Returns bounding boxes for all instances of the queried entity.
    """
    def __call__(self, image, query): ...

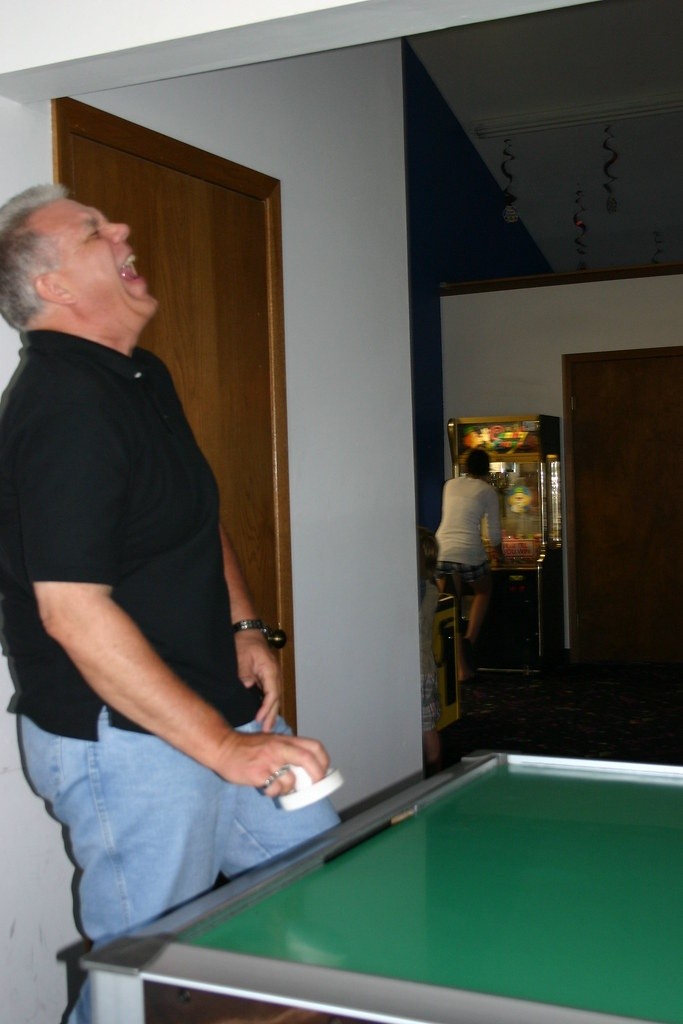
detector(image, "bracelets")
[232,619,263,634]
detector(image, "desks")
[78,747,683,1024]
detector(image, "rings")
[262,775,275,789]
[273,764,290,779]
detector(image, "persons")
[433,449,506,672]
[416,526,441,780]
[0,186,341,1024]
